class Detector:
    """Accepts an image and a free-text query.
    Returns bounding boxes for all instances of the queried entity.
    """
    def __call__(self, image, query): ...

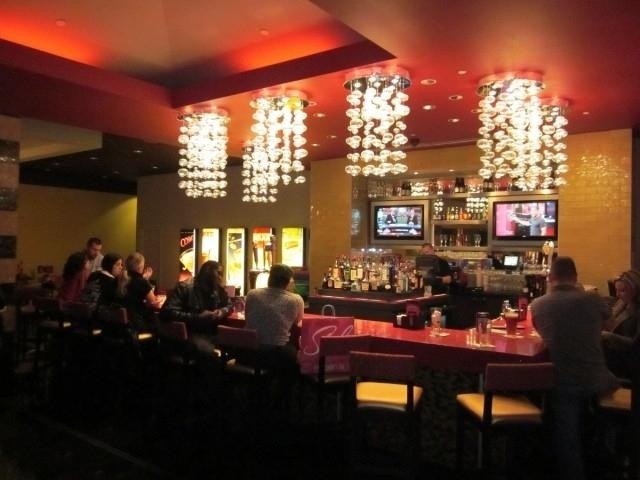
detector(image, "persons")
[530,255,612,479]
[602,267,637,385]
[70,253,124,331]
[246,263,305,366]
[82,237,105,274]
[529,208,543,235]
[386,209,398,232]
[417,243,454,293]
[161,261,235,367]
[111,251,161,334]
[407,208,417,231]
[54,255,91,313]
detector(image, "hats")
[613,269,640,299]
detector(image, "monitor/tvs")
[487,197,560,250]
[368,198,430,248]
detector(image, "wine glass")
[444,234,449,246]
[439,234,444,247]
[474,234,482,246]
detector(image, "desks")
[308,287,447,322]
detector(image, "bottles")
[455,206,459,220]
[460,207,463,218]
[323,255,423,292]
[401,181,410,195]
[455,224,466,247]
[445,206,450,220]
[450,207,454,220]
[502,300,511,312]
[454,177,465,193]
[464,207,483,220]
[483,176,495,192]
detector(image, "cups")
[505,313,518,335]
[430,307,442,333]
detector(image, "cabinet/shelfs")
[369,171,560,295]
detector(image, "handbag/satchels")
[301,305,355,377]
